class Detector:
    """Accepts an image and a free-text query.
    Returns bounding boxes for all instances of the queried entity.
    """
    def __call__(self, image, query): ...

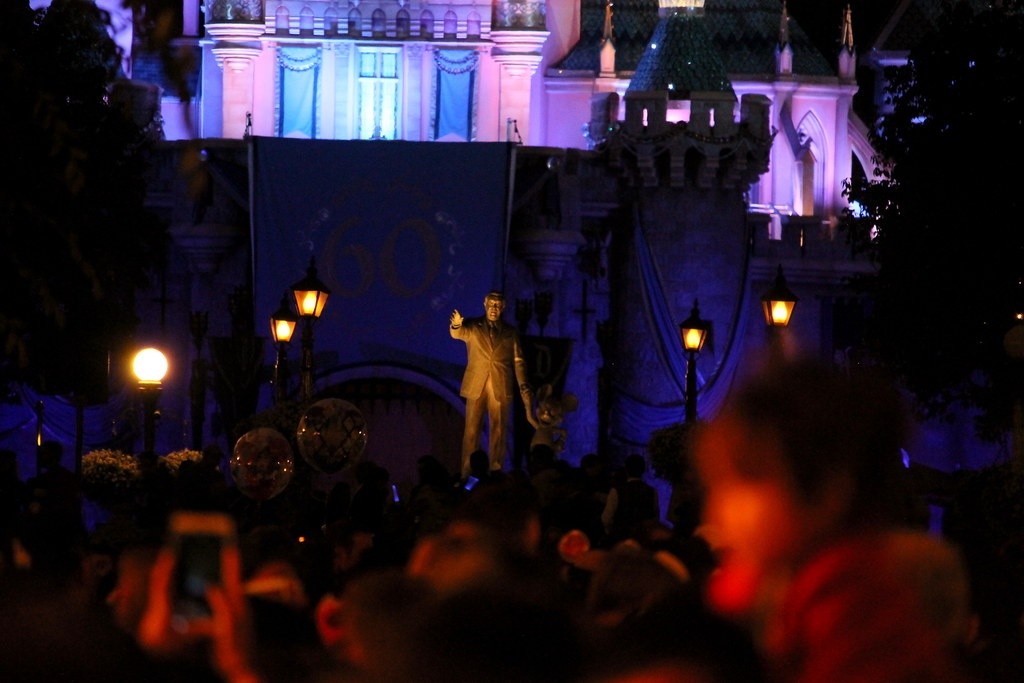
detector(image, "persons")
[448,292,533,480]
[3,353,1022,680]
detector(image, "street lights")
[759,267,795,365]
[679,298,714,424]
[296,273,328,405]
[134,346,169,490]
[269,299,299,423]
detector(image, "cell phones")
[169,511,234,642]
